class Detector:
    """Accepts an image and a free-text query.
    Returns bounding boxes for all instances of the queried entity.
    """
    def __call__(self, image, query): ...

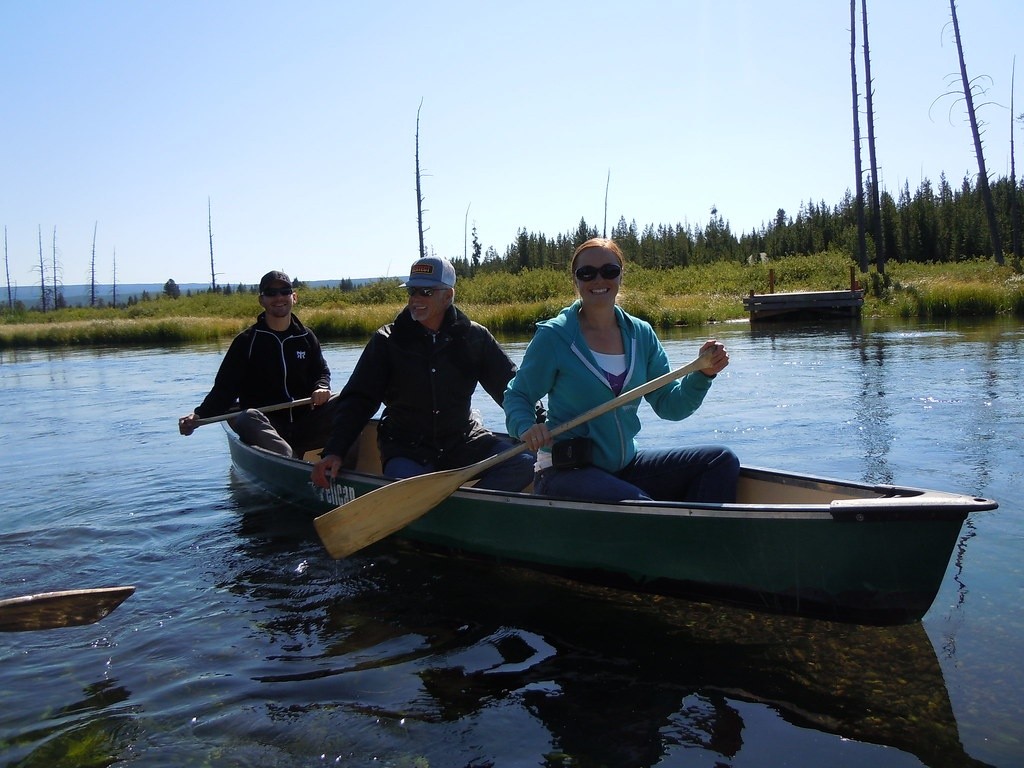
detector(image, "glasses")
[406,286,449,297]
[261,289,293,297]
[575,263,622,282]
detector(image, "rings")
[531,438,537,441]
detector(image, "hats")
[259,270,291,292]
[398,255,457,291]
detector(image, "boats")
[220,416,998,628]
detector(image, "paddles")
[185,394,335,427]
[312,345,719,560]
[0,587,134,632]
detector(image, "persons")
[178,270,343,459]
[312,256,546,493]
[502,239,741,503]
